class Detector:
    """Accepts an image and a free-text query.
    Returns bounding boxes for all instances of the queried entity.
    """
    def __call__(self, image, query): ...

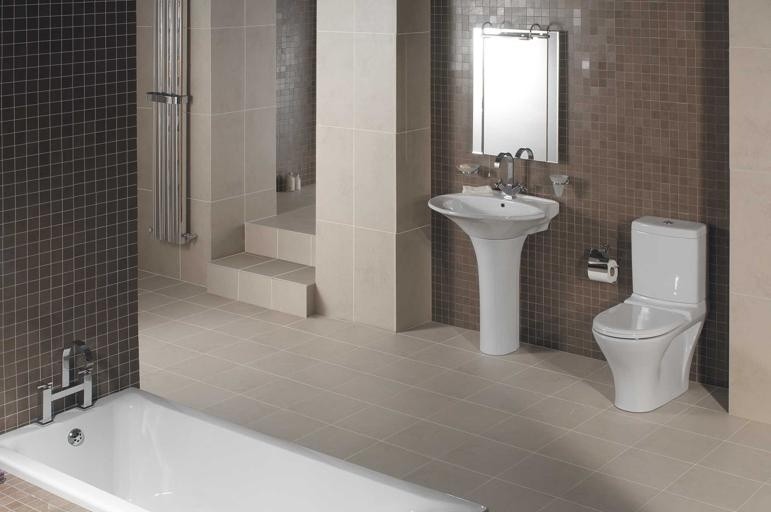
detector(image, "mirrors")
[471,26,561,165]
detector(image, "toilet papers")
[587,258,619,284]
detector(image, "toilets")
[591,214,712,413]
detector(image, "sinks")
[428,185,560,239]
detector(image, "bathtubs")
[0,386,489,512]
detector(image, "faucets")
[492,152,515,189]
[515,147,534,160]
[60,340,93,388]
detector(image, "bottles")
[285,171,302,192]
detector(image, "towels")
[462,185,492,195]
